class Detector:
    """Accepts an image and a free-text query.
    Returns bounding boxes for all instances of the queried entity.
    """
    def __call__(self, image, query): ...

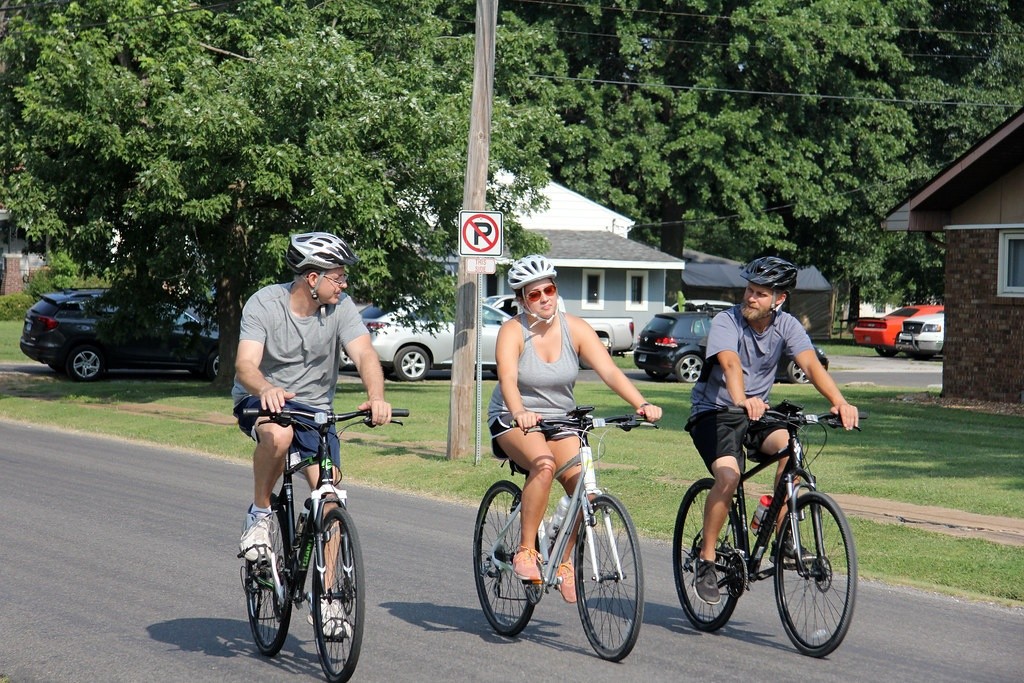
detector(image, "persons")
[232,232,392,639]
[486,254,663,603]
[683,257,859,605]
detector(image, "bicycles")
[238,401,410,683]
[473,406,659,661]
[673,407,866,658]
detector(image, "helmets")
[286,232,359,276]
[508,254,557,289]
[740,256,798,294]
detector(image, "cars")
[853,305,944,357]
[339,296,514,382]
[672,300,736,312]
[633,311,829,383]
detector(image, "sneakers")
[239,503,277,561]
[307,589,352,638]
[513,546,542,580]
[693,554,721,605]
[769,533,817,563]
[556,556,577,603]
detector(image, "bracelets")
[640,403,649,408]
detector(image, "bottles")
[545,495,572,539]
[750,495,773,536]
[293,513,308,549]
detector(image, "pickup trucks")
[482,295,634,369]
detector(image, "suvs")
[19,287,221,381]
[895,313,944,361]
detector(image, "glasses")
[526,285,556,301]
[317,273,348,287]
